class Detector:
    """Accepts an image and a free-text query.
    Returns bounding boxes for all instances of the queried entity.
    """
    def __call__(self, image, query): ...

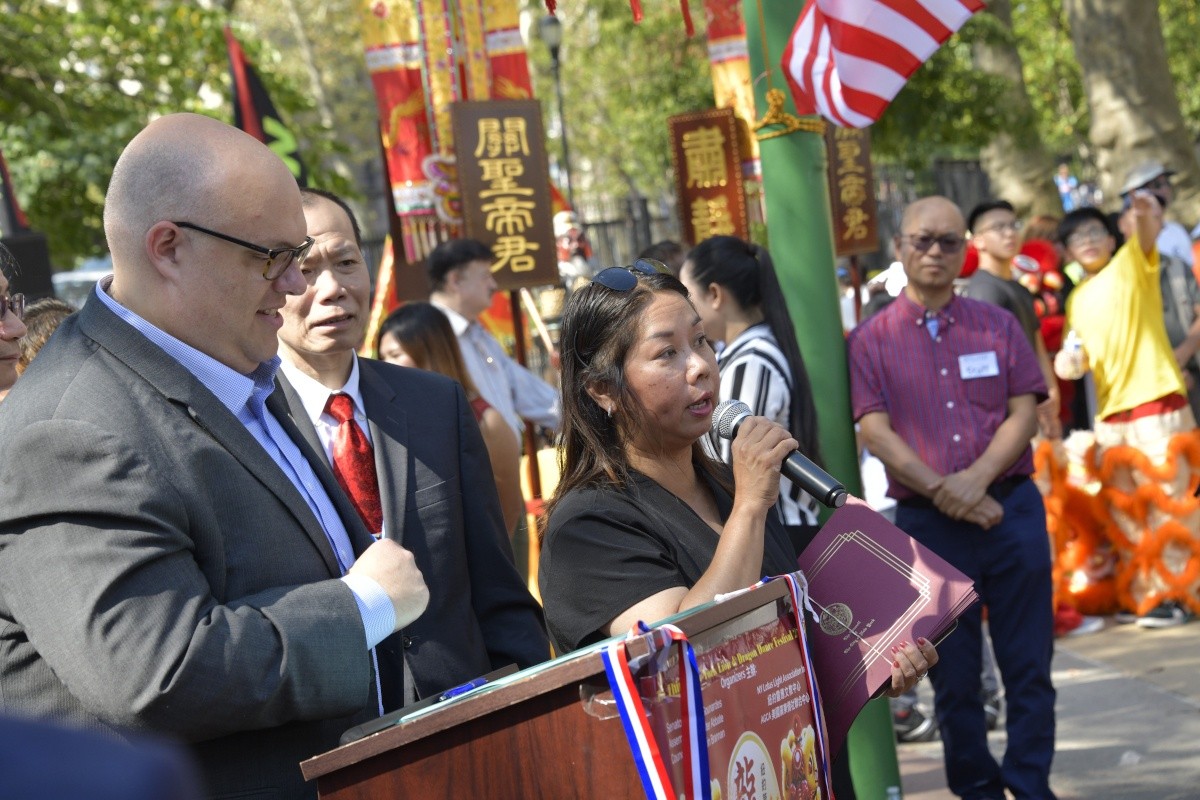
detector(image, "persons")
[379,239,564,541]
[275,185,551,722]
[838,154,1200,745]
[0,243,81,392]
[539,256,939,697]
[846,193,1062,799]
[639,235,827,558]
[553,210,594,290]
[1,109,433,800]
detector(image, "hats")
[1118,159,1175,197]
[967,200,1013,231]
[1057,205,1125,255]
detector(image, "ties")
[322,393,387,534]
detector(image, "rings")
[917,671,929,681]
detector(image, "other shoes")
[1115,604,1190,627]
[982,695,1001,729]
[1069,615,1106,636]
[892,704,936,741]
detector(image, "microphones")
[711,400,848,508]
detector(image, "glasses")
[901,233,967,254]
[979,218,1021,233]
[588,255,674,296]
[1071,225,1107,244]
[165,221,317,282]
